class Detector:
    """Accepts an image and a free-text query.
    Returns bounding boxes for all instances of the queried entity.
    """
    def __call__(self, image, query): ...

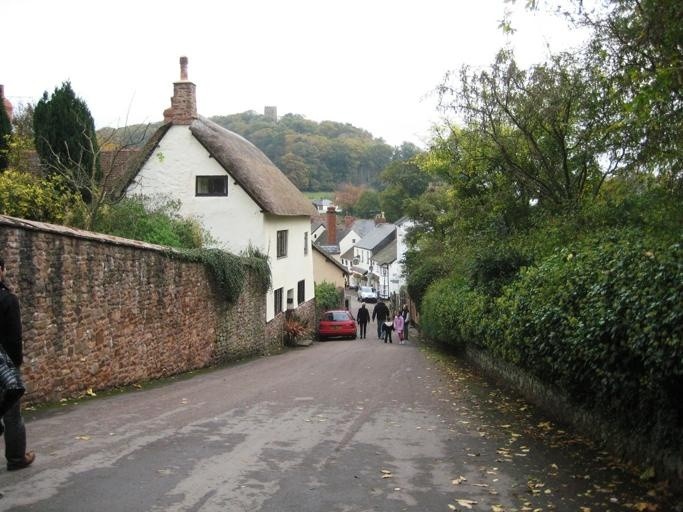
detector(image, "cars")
[318,309,357,340]
[357,285,378,303]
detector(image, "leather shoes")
[7,451,35,471]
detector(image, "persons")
[393,310,404,345]
[381,317,394,343]
[371,299,389,340]
[0,253,35,471]
[357,301,370,339]
[400,304,410,340]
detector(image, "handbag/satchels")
[0,345,26,418]
[357,319,360,324]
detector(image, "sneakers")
[399,341,405,344]
